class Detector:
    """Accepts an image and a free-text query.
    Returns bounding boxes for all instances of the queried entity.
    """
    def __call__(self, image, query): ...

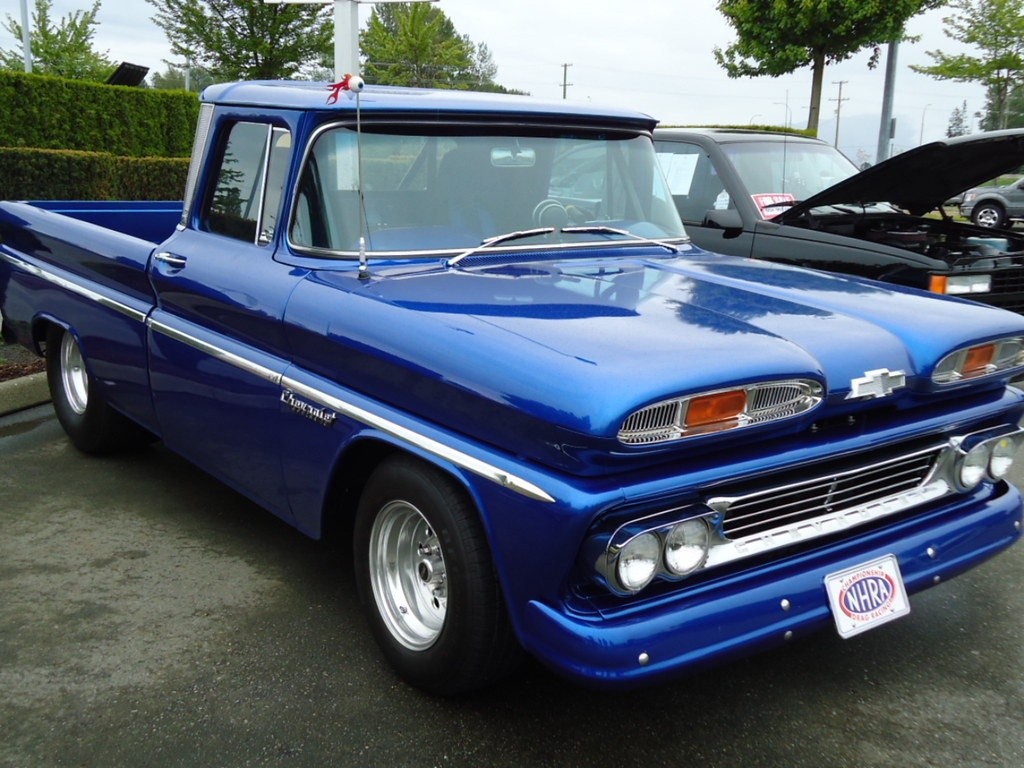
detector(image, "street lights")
[774,101,793,127]
[749,114,762,126]
[919,104,933,145]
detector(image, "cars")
[960,177,1024,228]
[544,125,1024,315]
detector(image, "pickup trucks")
[1,77,1024,706]
